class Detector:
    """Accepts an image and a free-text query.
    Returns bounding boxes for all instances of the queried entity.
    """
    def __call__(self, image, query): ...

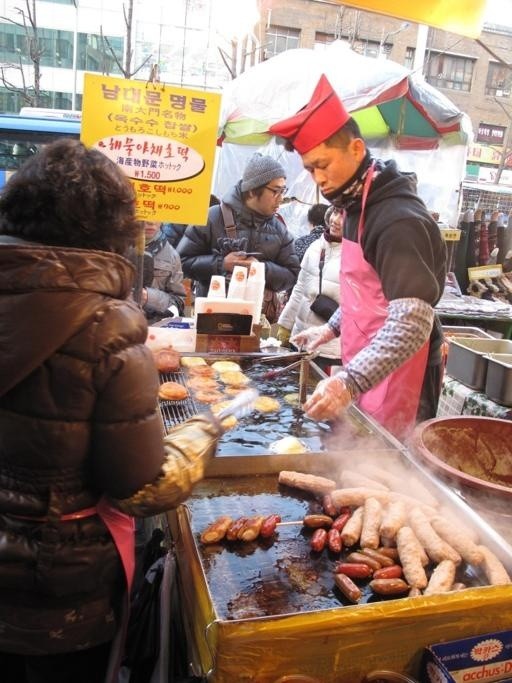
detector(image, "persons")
[274,202,350,374]
[159,193,223,247]
[269,71,449,444]
[0,133,226,683]
[174,152,301,318]
[140,219,187,326]
[283,203,332,306]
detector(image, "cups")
[226,261,265,323]
[208,275,226,297]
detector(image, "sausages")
[198,468,512,600]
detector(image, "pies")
[158,356,312,455]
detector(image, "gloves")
[219,237,248,281]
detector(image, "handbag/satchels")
[311,293,338,321]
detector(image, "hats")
[241,152,286,192]
[269,74,353,155]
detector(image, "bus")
[0,107,83,194]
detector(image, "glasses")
[265,187,286,199]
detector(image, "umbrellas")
[211,37,475,205]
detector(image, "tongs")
[251,350,321,380]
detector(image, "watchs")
[337,367,362,401]
